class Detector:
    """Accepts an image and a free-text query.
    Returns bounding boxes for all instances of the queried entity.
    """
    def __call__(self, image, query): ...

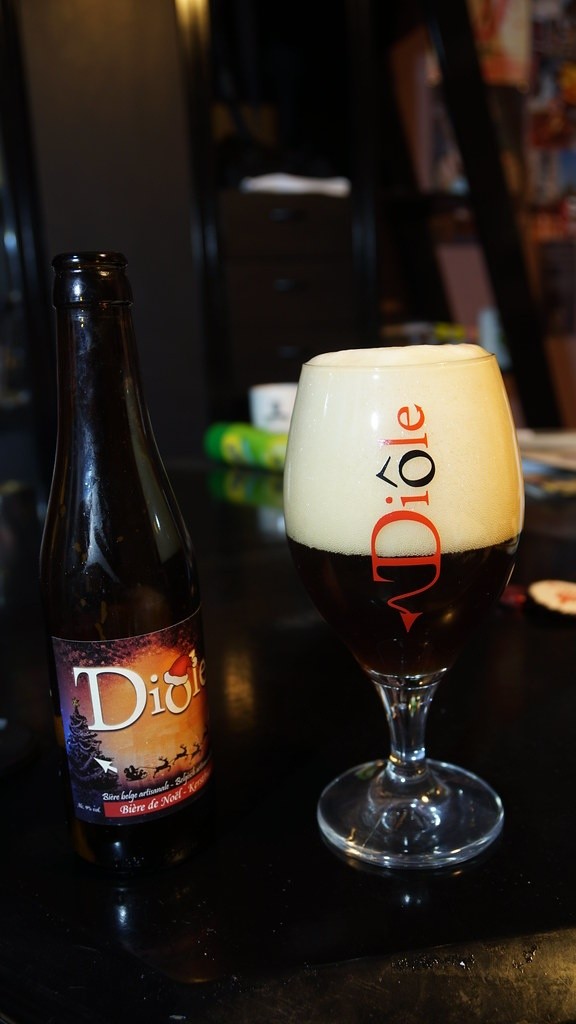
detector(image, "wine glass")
[284,353,526,866]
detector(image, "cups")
[252,383,297,434]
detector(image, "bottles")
[36,246,221,877]
